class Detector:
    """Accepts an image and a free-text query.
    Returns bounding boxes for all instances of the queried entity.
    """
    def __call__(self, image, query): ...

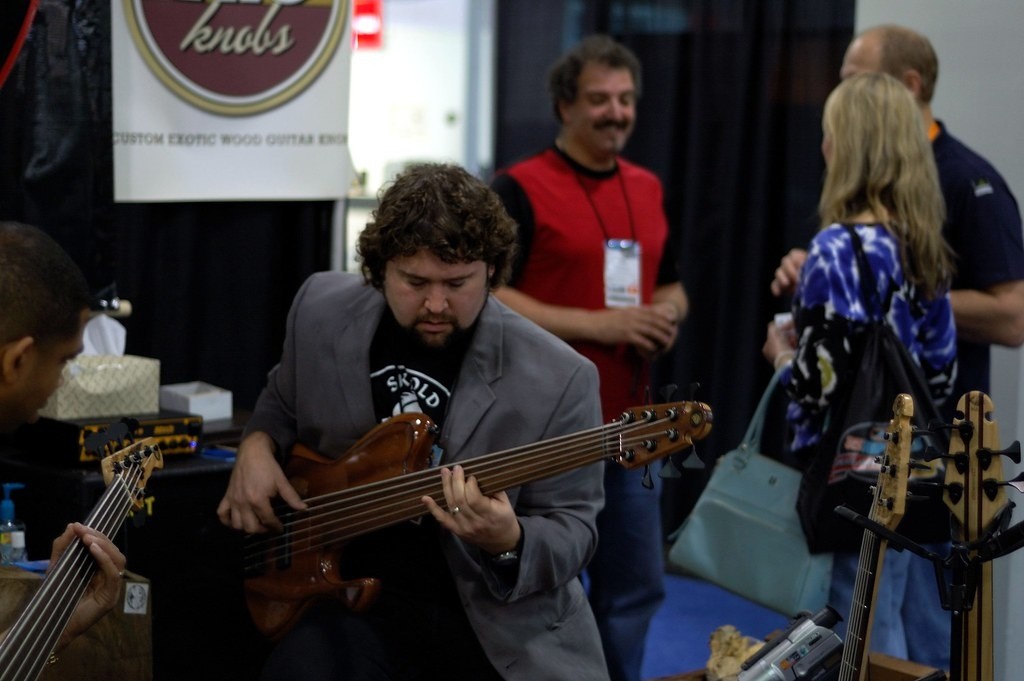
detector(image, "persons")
[760,25,1024,669]
[0,220,127,669]
[489,32,690,681]
[216,163,607,681]
[759,73,960,681]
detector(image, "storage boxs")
[159,382,233,421]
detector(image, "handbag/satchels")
[666,353,834,619]
[793,220,956,557]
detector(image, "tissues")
[37,312,161,421]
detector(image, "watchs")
[498,551,519,565]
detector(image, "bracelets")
[49,651,58,663]
[772,351,795,370]
[653,297,681,321]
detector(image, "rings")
[118,570,125,575]
[449,506,460,515]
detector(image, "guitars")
[841,391,1019,677]
[243,385,714,639]
[0,437,163,681]
